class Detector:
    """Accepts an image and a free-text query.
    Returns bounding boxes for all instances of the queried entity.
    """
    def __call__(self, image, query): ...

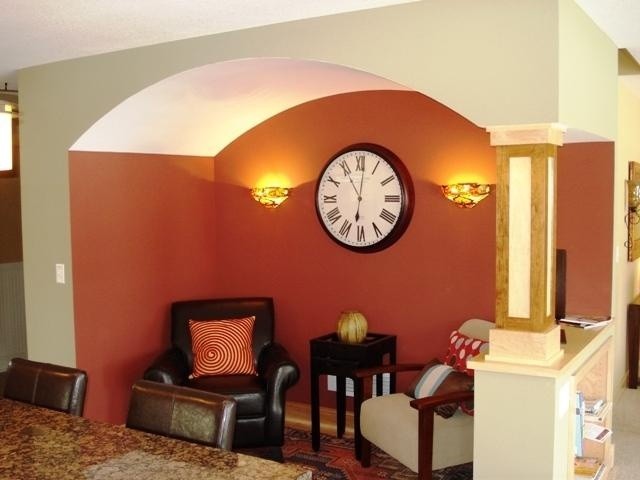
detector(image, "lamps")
[249,186,289,209]
[441,182,491,209]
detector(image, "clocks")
[314,147,406,251]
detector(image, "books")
[574,376,613,480]
[559,314,612,330]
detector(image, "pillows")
[405,328,490,420]
[188,315,259,379]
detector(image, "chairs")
[352,317,496,479]
[143,296,301,450]
